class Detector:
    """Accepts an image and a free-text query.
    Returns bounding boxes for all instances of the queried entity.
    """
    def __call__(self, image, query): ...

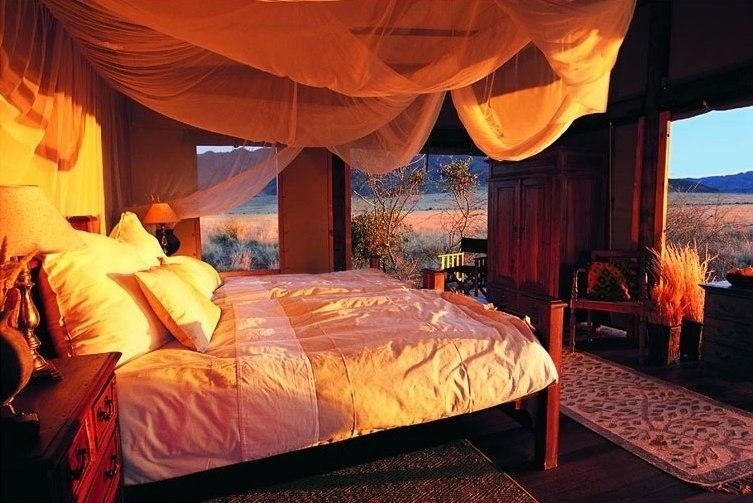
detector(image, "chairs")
[434,251,487,298]
[569,248,651,367]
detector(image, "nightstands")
[1,351,125,503]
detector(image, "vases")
[666,315,683,370]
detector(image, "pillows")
[585,260,631,300]
[42,211,224,369]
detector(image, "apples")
[743,267,753,276]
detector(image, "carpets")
[201,438,541,503]
[541,341,753,496]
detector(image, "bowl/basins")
[724,272,753,289]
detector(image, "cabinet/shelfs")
[697,281,753,388]
[483,144,603,349]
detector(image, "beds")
[26,216,568,470]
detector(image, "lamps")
[0,184,89,384]
[141,202,179,255]
